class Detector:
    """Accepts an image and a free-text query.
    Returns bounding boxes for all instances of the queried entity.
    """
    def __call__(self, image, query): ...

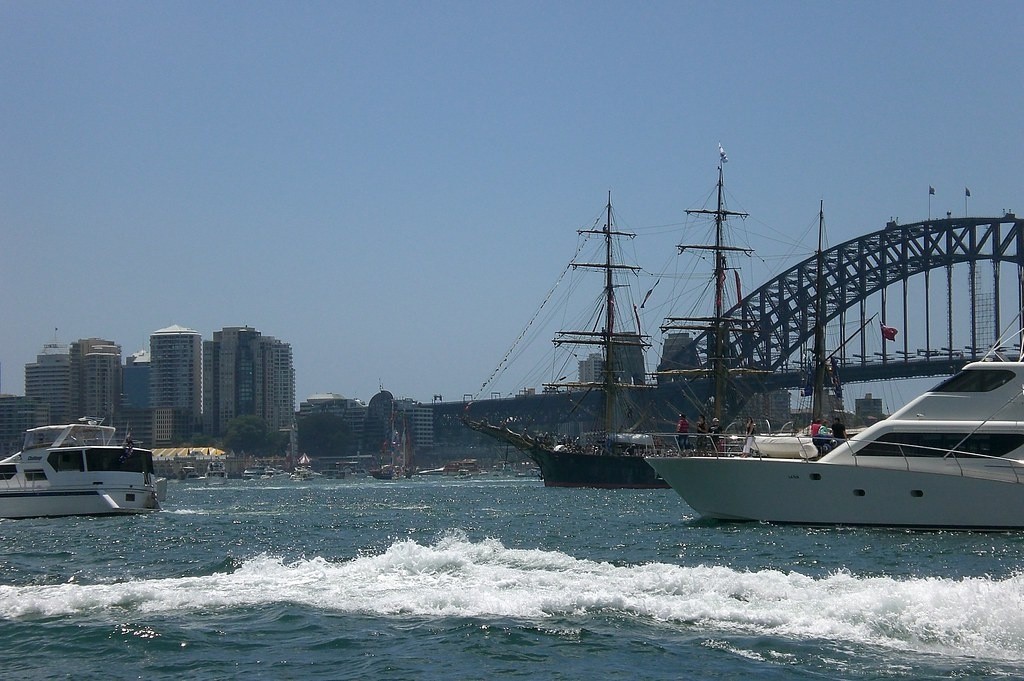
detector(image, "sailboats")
[581,140,757,456]
[461,190,849,490]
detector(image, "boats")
[205,455,228,479]
[454,469,472,479]
[290,466,323,482]
[369,465,405,479]
[178,461,199,480]
[0,415,167,518]
[242,465,290,480]
[644,309,1024,533]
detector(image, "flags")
[966,188,970,196]
[929,187,935,195]
[881,324,898,342]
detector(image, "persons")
[812,419,829,435]
[696,414,708,457]
[677,413,690,457]
[741,417,754,458]
[707,418,724,457]
[831,417,847,439]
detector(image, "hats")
[713,418,719,422]
